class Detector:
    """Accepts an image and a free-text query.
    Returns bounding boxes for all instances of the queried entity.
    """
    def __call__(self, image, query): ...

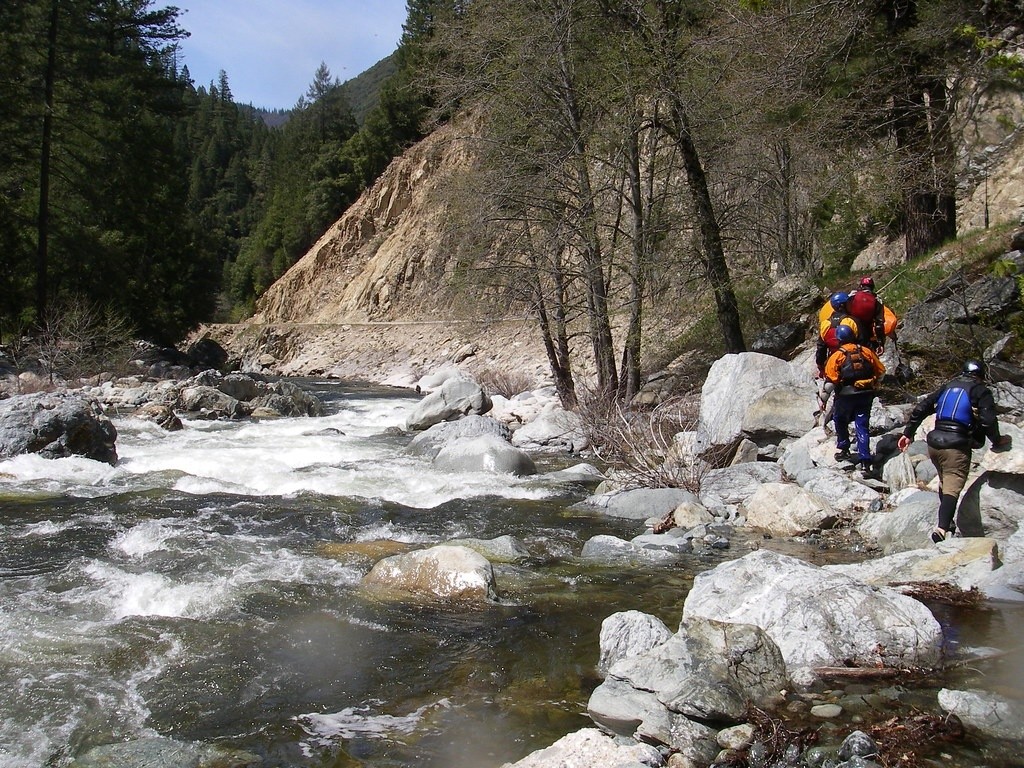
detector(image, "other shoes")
[948,521,955,529]
[932,530,945,544]
[834,449,851,462]
[860,462,872,478]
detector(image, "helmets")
[961,358,985,380]
[860,277,874,286]
[835,325,855,342]
[830,292,852,307]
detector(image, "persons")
[899,356,1010,543]
[819,325,886,478]
[815,291,863,380]
[843,278,896,356]
